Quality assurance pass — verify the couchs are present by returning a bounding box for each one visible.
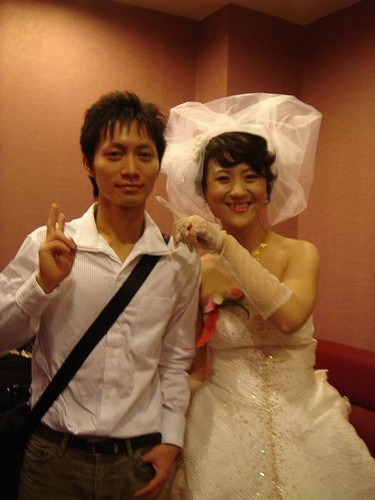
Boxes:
[314,339,375,456]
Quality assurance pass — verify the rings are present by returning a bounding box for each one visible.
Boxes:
[186,222,193,231]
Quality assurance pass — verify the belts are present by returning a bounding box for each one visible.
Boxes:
[32,423,162,455]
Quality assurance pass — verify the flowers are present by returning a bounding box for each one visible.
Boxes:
[194,287,250,347]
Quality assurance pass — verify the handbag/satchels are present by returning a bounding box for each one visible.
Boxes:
[0,352,32,500]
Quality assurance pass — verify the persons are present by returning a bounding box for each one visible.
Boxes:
[0,89,203,500]
[156,131,375,500]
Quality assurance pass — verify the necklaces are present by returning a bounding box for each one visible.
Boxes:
[251,230,272,261]
